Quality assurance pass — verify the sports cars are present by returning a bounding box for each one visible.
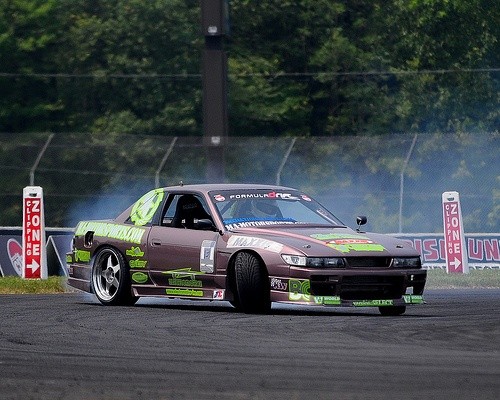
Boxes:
[67,182,427,316]
